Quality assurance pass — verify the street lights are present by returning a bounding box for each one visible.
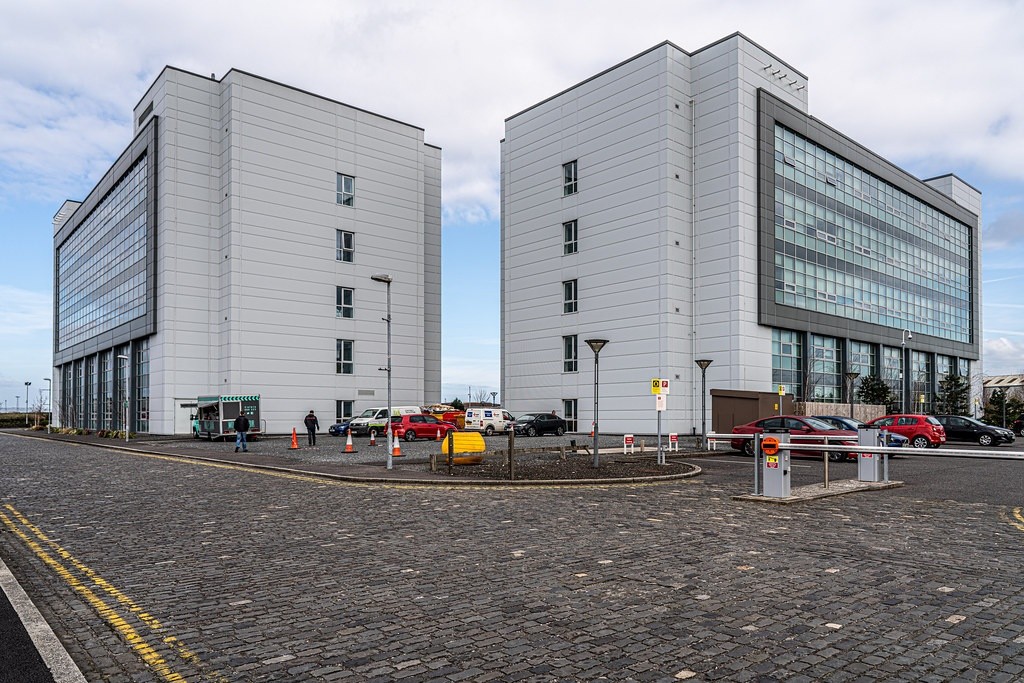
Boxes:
[371,274,394,469]
[117,354,129,442]
[695,360,713,450]
[584,340,609,469]
[15,378,51,434]
[491,392,498,407]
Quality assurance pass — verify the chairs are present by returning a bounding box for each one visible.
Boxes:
[795,422,802,430]
[899,419,904,425]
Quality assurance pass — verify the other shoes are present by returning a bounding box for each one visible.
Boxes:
[244,449,248,452]
[235,447,239,453]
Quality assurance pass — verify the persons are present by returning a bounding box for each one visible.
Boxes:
[234,411,250,453]
[304,410,319,446]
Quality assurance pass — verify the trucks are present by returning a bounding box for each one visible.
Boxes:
[190,394,261,442]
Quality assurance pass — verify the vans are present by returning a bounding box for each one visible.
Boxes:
[349,406,421,438]
[464,409,515,437]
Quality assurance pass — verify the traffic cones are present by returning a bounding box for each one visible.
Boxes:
[341,429,377,454]
[287,427,300,450]
[392,430,406,457]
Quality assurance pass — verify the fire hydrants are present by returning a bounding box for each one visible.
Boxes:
[901,329,913,414]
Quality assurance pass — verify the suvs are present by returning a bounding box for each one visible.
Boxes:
[384,414,458,441]
[1014,413,1024,437]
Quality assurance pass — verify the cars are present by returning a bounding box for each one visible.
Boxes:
[731,415,859,463]
[504,413,566,437]
[329,415,358,437]
[936,415,1016,447]
[812,415,946,458]
